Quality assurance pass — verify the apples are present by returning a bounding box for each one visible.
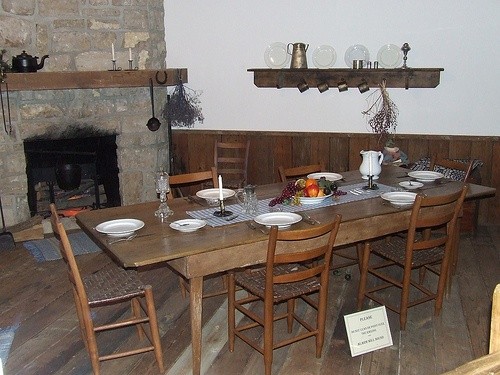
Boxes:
[302,178,324,197]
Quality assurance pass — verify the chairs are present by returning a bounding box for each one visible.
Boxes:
[164,141,474,375]
[48,203,165,375]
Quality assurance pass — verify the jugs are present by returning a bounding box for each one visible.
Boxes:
[359,150,383,180]
[235,184,258,215]
[286,43,310,69]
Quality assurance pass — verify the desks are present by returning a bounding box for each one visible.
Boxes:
[75,163,495,375]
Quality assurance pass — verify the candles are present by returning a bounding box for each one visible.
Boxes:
[112,43,115,60]
[128,47,132,60]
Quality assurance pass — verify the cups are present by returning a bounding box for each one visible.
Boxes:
[352,60,363,69]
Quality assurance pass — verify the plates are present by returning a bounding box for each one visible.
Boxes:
[195,188,235,199]
[377,44,403,69]
[95,218,145,237]
[264,42,290,69]
[307,172,343,182]
[291,190,333,204]
[169,218,207,232]
[398,180,424,190]
[344,44,369,69]
[254,212,302,229]
[408,170,444,182]
[312,45,336,69]
[381,191,417,205]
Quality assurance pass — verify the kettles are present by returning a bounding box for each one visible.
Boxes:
[11,51,49,73]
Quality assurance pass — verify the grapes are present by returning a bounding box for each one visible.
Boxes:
[335,190,347,196]
[268,182,303,206]
